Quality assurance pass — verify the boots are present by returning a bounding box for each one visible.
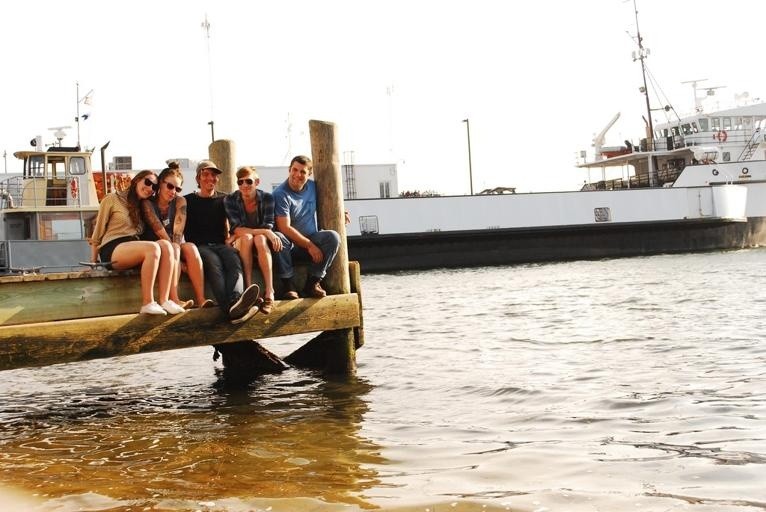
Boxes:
[303,274,327,297]
[280,279,298,300]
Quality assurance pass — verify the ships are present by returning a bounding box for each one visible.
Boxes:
[0,1,766,274]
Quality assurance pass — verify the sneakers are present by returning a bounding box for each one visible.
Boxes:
[177,300,194,309]
[161,300,185,315]
[226,283,264,324]
[199,299,215,308]
[140,302,168,316]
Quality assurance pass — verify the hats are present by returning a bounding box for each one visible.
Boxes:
[196,160,223,175]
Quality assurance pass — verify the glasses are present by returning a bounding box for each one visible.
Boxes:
[163,180,182,192]
[144,177,159,191]
[237,179,255,185]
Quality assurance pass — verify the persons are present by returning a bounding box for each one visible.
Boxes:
[181,162,262,325]
[271,155,350,300]
[140,161,214,310]
[89,171,184,316]
[223,167,282,314]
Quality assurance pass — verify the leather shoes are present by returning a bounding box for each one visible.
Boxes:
[260,297,274,314]
